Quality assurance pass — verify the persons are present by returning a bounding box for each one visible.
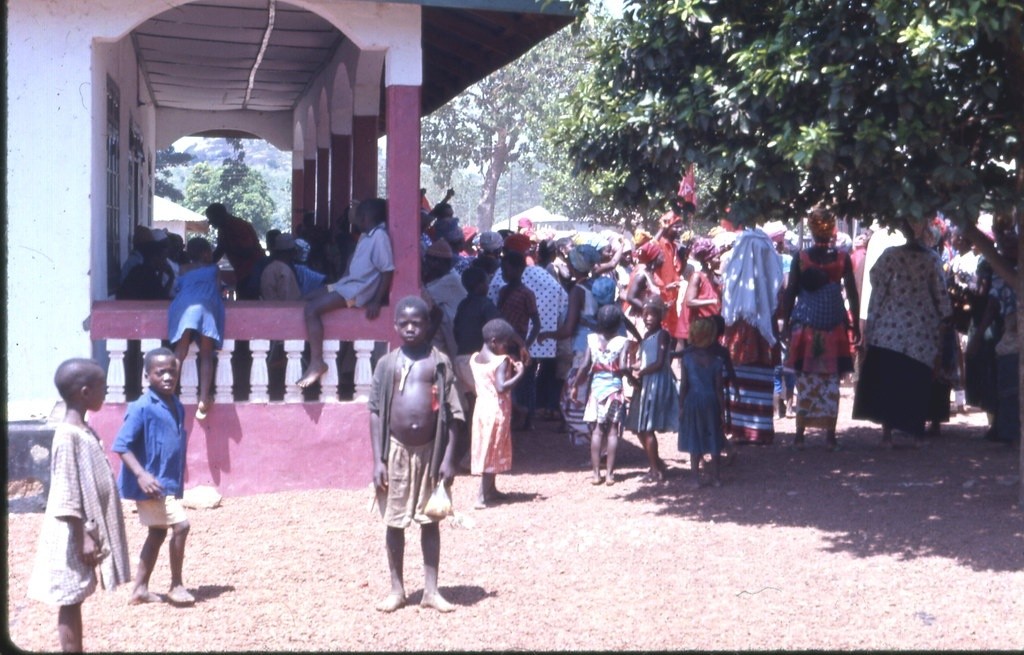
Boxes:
[630,295,673,483]
[120,197,396,384]
[167,237,227,420]
[369,295,466,614]
[420,188,1024,467]
[26,358,131,654]
[569,304,640,485]
[671,315,736,491]
[111,348,195,606]
[461,320,525,507]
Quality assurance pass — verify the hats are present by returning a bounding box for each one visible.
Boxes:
[690,317,717,348]
[643,295,665,311]
[427,237,453,258]
[592,276,616,306]
[570,245,601,273]
[807,210,836,236]
[504,234,531,255]
[693,238,718,262]
[481,232,503,252]
